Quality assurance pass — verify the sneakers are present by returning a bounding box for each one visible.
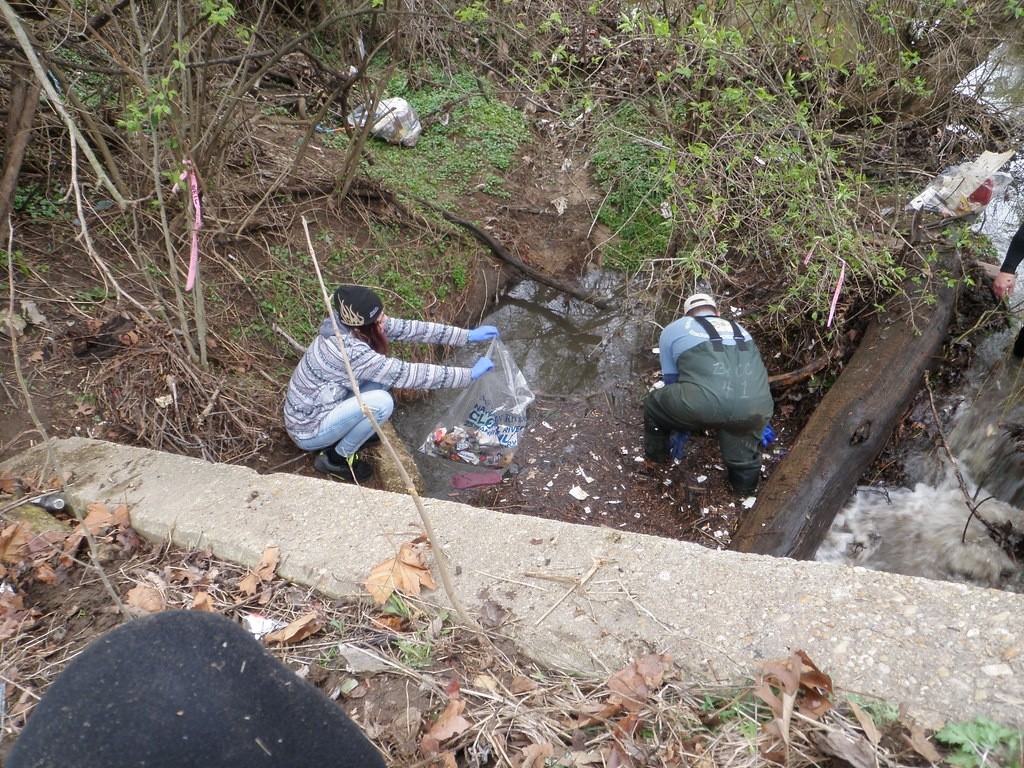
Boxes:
[366,432,380,443]
[314,449,373,483]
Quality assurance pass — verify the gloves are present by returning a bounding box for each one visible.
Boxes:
[471,357,493,380]
[467,325,500,342]
[761,424,775,447]
[664,373,690,460]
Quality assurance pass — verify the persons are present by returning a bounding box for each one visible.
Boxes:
[283,285,500,486]
[992,221,1024,360]
[642,293,774,499]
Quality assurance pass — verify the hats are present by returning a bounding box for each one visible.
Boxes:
[334,285,383,327]
[684,294,718,317]
